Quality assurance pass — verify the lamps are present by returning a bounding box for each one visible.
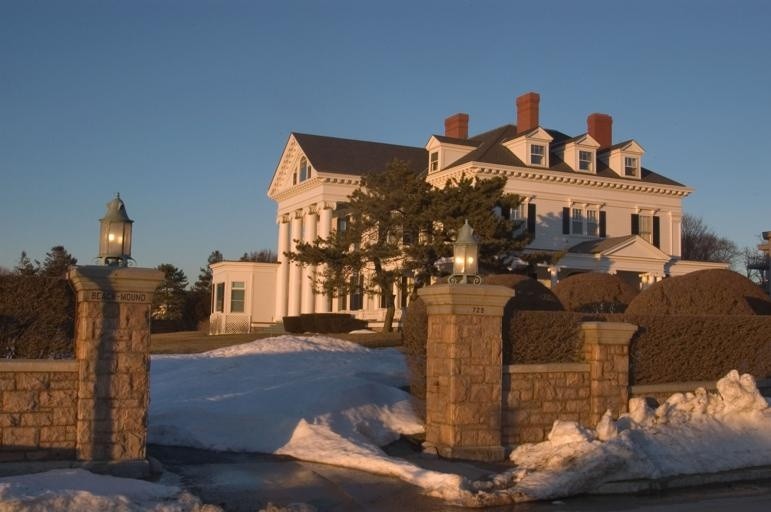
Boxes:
[95,189,137,268]
[447,220,484,287]
